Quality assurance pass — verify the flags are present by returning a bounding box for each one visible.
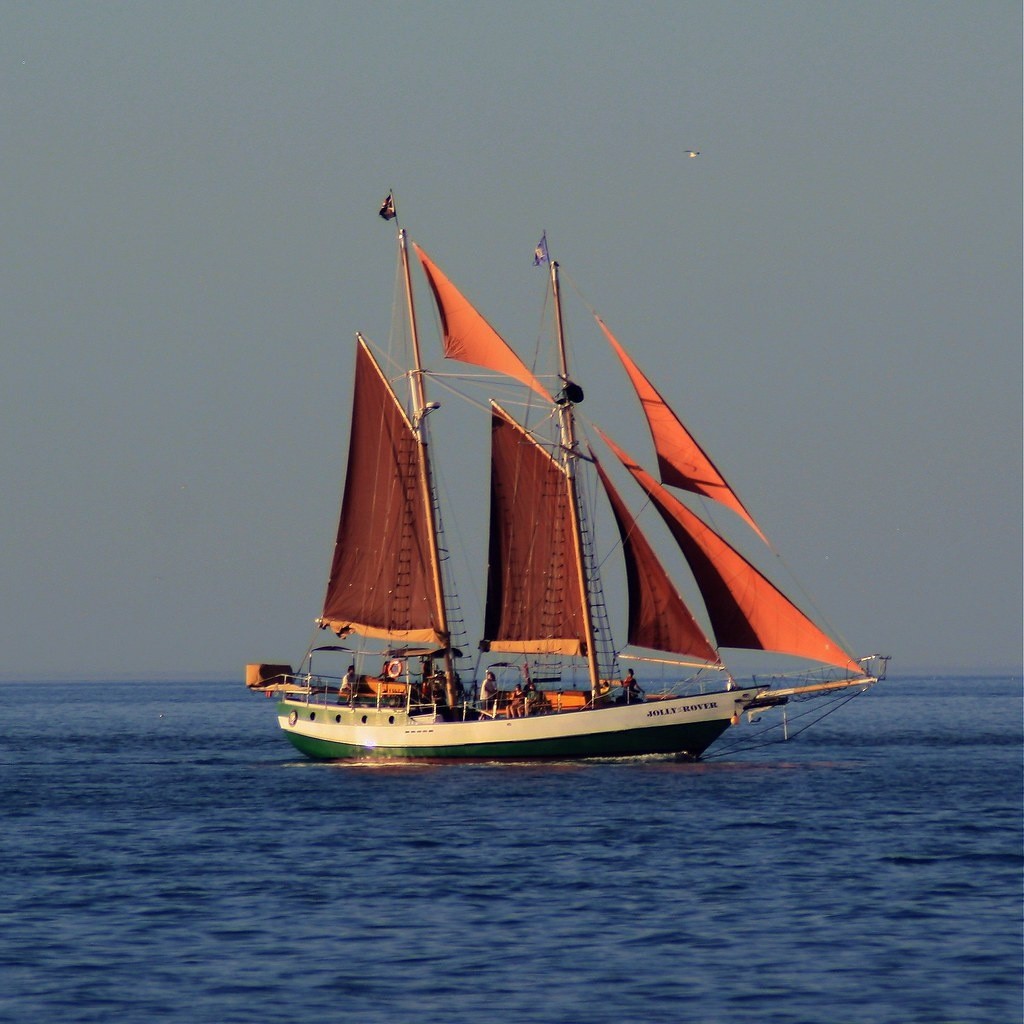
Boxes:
[378,193,398,220]
[533,235,547,266]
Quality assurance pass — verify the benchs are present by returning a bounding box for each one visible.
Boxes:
[479,707,524,719]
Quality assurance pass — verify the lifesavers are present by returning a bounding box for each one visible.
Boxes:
[387,659,403,678]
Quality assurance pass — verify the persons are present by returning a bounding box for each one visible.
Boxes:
[429,672,547,721]
[623,669,646,705]
[338,665,358,708]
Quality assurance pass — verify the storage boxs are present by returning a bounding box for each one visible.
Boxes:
[246,664,293,687]
[544,690,590,711]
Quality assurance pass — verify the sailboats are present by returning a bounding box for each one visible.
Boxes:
[243,184,888,766]
